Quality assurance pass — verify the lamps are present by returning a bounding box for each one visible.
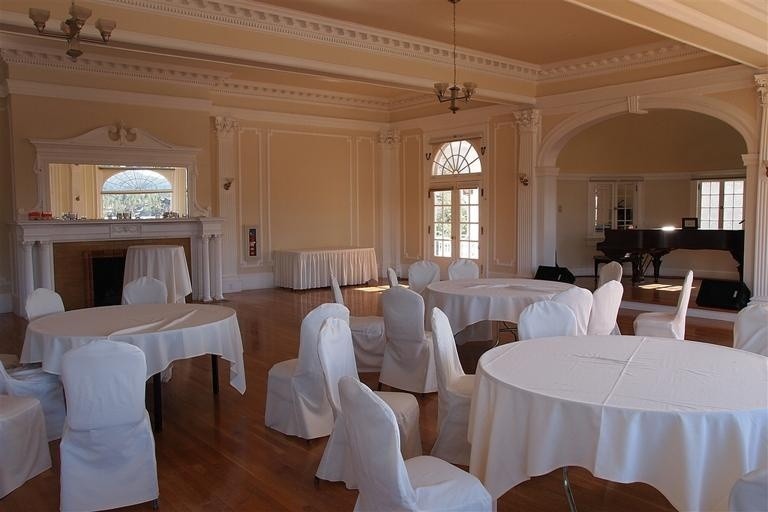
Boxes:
[223,176,235,190]
[28,0,117,63]
[431,0,478,115]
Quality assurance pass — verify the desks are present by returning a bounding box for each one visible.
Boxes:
[272,246,379,290]
[18,303,247,435]
[420,275,597,353]
[471,334,765,510]
[9,219,224,318]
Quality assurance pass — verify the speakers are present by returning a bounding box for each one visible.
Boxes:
[534,265,575,284]
[696,278,751,311]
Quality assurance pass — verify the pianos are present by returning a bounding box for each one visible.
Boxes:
[597,228,744,283]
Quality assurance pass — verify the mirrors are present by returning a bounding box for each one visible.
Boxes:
[49,163,189,220]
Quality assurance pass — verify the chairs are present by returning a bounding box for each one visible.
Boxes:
[120,275,171,305]
[428,304,481,471]
[591,278,625,336]
[336,372,493,510]
[323,260,390,379]
[383,266,399,290]
[0,393,54,500]
[731,303,767,357]
[314,316,424,490]
[405,260,443,294]
[448,258,478,282]
[264,302,352,444]
[56,340,164,512]
[24,287,67,321]
[375,282,439,402]
[547,284,595,337]
[1,355,67,446]
[514,299,579,340]
[631,267,697,342]
[598,260,625,336]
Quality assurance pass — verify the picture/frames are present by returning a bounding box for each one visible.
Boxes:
[682,217,698,230]
